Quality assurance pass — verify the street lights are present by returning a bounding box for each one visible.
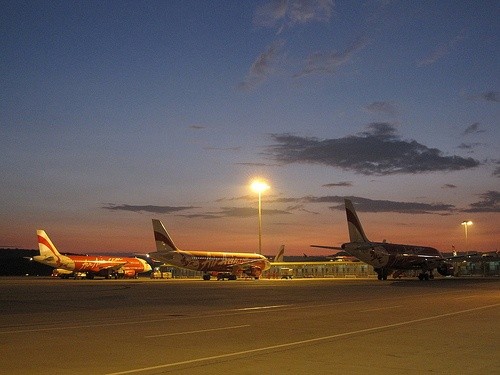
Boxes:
[250,179,267,254]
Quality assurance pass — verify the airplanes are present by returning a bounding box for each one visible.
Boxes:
[309,197,445,281]
[23,229,153,280]
[147,219,271,280]
